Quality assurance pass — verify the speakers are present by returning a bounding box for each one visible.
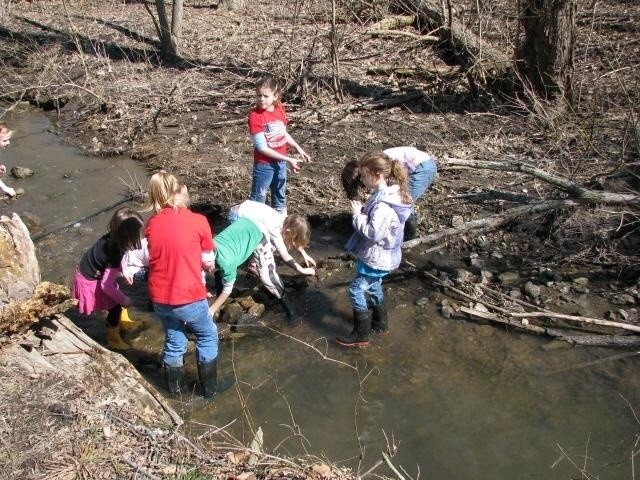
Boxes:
[197,355,236,398]
[161,358,197,394]
[278,288,304,330]
[403,214,419,244]
[119,307,143,330]
[104,324,131,351]
[335,303,390,348]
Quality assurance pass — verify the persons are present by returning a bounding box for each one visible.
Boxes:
[224,199,319,277]
[142,169,238,399]
[70,206,146,352]
[207,217,301,329]
[245,74,312,217]
[339,144,438,241]
[119,236,217,300]
[335,149,417,350]
